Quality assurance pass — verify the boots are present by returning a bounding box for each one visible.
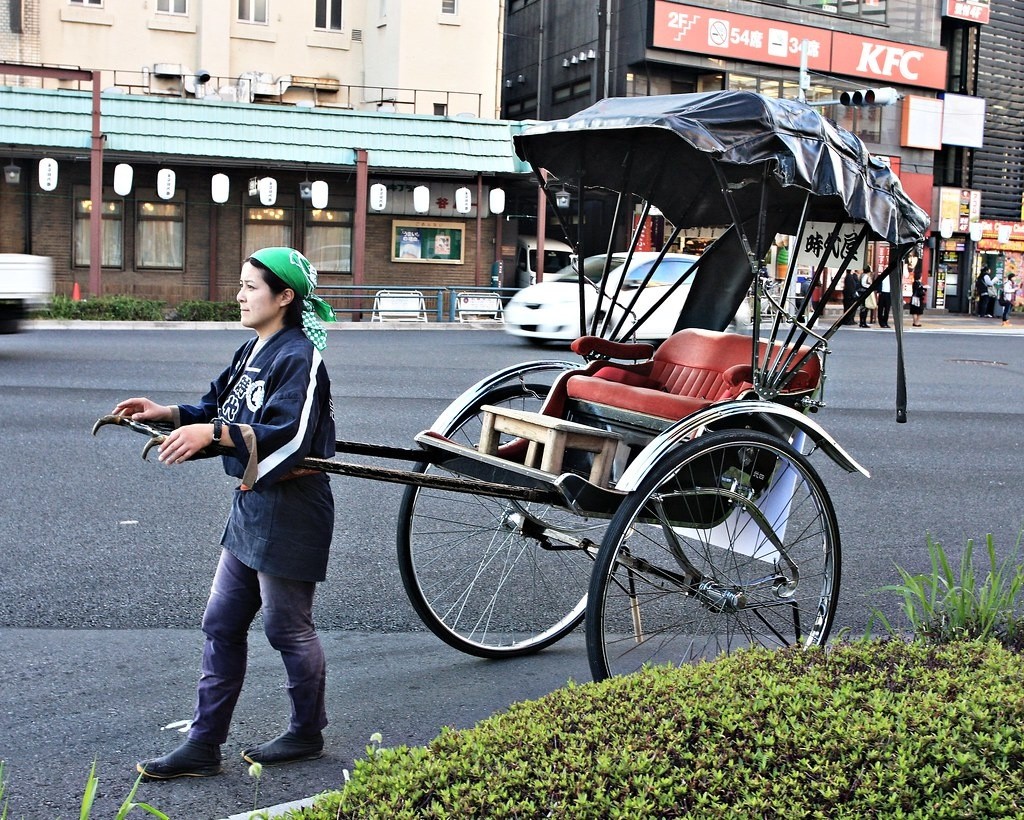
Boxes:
[859,311,870,327]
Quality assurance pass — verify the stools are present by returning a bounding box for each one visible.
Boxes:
[478,405,623,488]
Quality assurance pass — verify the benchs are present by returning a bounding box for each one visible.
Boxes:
[565,327,820,432]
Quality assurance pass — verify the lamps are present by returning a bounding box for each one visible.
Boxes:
[555,184,570,208]
[247,164,262,197]
[299,162,313,200]
[4,146,21,184]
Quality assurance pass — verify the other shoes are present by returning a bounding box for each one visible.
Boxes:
[242,730,324,766]
[913,323,921,326]
[880,323,890,328]
[137,738,224,778]
[984,314,992,317]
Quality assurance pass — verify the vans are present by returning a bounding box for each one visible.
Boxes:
[503,251,751,344]
[515,235,579,291]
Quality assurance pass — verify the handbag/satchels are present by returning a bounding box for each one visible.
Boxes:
[988,287,997,298]
[865,291,877,309]
[912,297,920,307]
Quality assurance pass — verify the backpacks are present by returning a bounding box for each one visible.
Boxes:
[998,282,1007,306]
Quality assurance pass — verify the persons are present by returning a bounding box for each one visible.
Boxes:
[112,246,337,776]
[811,280,825,318]
[910,271,928,326]
[905,244,920,272]
[843,265,891,328]
[976,266,1018,327]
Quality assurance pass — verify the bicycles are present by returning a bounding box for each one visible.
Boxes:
[739,281,798,326]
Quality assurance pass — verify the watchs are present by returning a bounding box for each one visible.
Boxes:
[209,418,222,445]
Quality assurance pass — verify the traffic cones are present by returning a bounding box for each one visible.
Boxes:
[72,283,80,300]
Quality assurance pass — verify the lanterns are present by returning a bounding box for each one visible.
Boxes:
[970,222,983,242]
[157,169,176,199]
[489,188,505,215]
[212,173,229,205]
[39,158,58,191]
[370,183,387,212]
[259,177,277,205]
[997,224,1012,245]
[455,187,472,214]
[114,163,133,197]
[940,218,954,239]
[311,181,328,209]
[413,185,429,214]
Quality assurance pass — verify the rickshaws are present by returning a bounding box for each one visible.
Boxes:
[92,91,928,685]
